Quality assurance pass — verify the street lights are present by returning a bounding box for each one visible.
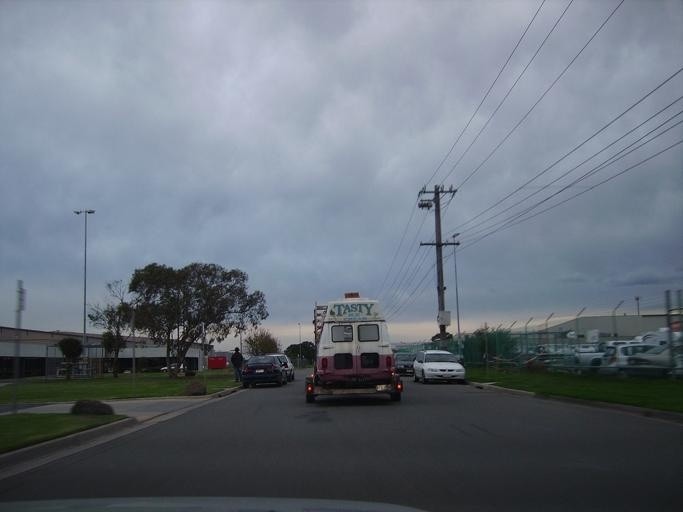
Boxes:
[297,322,301,368]
[452,233,463,364]
[631,297,640,317]
[73,209,94,376]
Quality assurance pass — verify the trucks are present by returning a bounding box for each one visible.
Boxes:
[207,356,229,369]
[304,292,402,401]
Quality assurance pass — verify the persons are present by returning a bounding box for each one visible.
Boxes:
[230,347,245,381]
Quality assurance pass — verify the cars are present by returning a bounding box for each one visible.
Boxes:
[413,350,466,384]
[263,353,294,382]
[393,352,415,377]
[510,327,683,380]
[159,363,187,372]
[240,355,287,388]
